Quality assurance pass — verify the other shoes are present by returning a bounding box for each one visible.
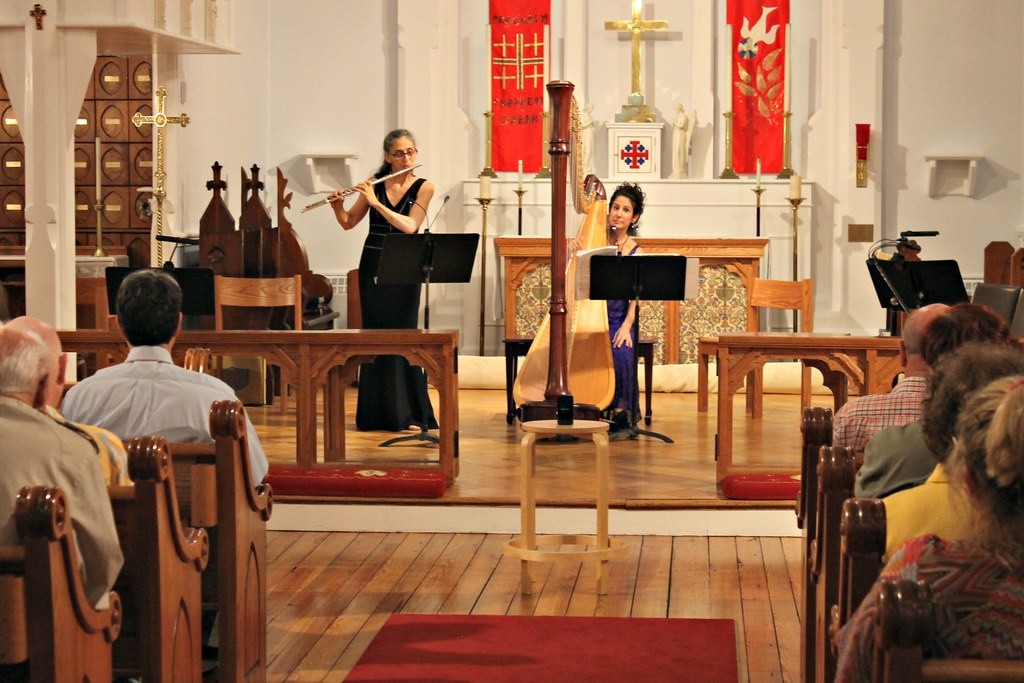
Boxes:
[611,413,631,432]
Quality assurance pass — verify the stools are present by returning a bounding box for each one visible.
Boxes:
[501,419,632,596]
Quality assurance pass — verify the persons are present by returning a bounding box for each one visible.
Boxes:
[0,316,135,612]
[60,271,269,488]
[833,303,1024,683]
[567,181,647,432]
[330,128,440,432]
[668,103,689,177]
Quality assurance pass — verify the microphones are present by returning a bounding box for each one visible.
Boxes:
[901,231,939,237]
[157,235,200,245]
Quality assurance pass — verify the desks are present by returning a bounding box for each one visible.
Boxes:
[0,254,129,319]
[715,329,906,486]
[55,328,461,489]
[492,237,770,364]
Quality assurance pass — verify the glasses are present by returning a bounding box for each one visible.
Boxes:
[388,148,417,158]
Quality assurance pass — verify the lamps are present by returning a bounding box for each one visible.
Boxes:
[855,122,872,188]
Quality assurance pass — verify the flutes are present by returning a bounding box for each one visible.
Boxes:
[299,162,423,214]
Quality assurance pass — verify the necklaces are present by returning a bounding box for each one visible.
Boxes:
[608,234,629,255]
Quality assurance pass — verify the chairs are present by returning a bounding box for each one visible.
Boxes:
[696,277,814,420]
[971,282,1020,325]
[1010,287,1024,336]
[214,274,302,417]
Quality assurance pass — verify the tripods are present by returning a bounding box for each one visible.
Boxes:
[589,255,687,444]
[379,233,480,447]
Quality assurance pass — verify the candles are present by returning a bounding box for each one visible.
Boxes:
[789,172,802,199]
[542,24,551,112]
[95,135,102,200]
[725,23,733,113]
[756,158,761,190]
[518,159,523,191]
[485,23,493,112]
[479,175,491,198]
[783,21,792,115]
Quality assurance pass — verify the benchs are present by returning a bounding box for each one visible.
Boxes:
[828,497,887,659]
[123,400,274,683]
[866,577,1024,683]
[807,443,856,683]
[501,333,658,426]
[795,406,863,683]
[107,434,210,683]
[0,485,122,683]
